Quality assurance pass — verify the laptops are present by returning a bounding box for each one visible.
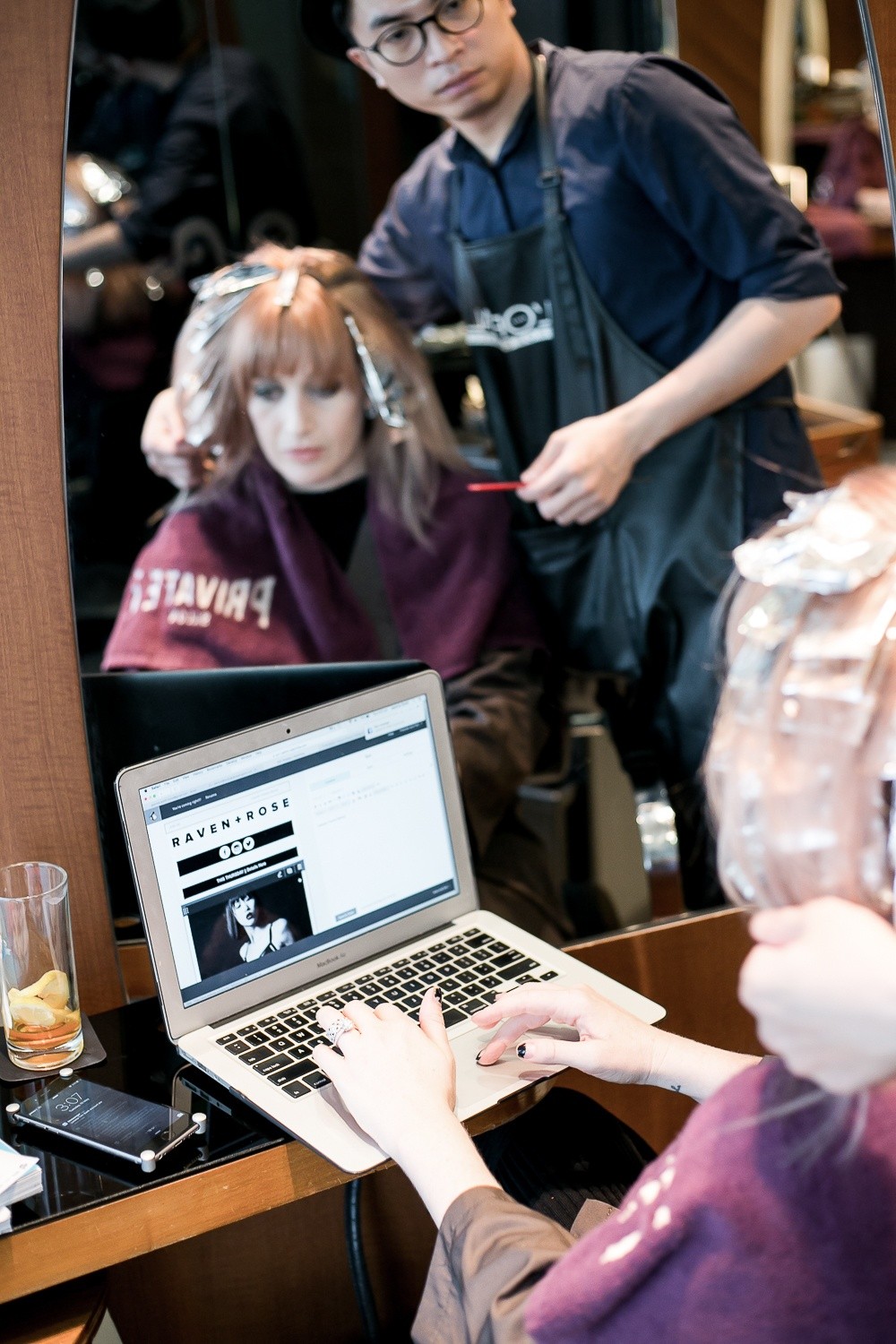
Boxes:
[116,671,670,1174]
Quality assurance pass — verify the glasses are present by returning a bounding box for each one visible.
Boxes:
[356,0,486,68]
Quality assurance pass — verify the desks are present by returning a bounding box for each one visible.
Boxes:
[0,997,560,1344]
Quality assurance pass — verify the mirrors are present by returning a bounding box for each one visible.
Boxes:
[0,0,896,1013]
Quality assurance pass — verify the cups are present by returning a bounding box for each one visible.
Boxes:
[1,860,84,1072]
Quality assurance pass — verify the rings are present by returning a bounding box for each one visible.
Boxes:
[328,1018,358,1047]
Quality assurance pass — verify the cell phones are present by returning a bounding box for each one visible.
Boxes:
[14,1075,200,1164]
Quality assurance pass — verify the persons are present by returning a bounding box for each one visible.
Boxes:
[313,466,896,1344]
[59,0,302,283]
[100,242,571,947]
[813,51,893,228]
[343,0,845,915]
[225,891,295,962]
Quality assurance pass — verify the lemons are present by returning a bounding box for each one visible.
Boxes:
[9,996,55,1027]
